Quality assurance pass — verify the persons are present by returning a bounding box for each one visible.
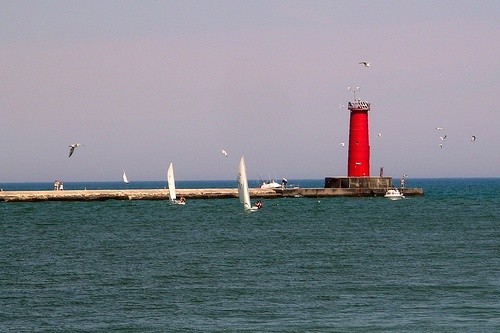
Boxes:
[177,196,184,202]
[399,176,405,188]
[54,180,64,191]
[255,199,261,207]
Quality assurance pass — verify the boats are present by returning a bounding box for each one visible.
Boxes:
[260,179,282,189]
[384,190,405,200]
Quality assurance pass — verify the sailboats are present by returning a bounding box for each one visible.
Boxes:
[167,161,187,205]
[237,155,259,213]
[122,168,130,184]
